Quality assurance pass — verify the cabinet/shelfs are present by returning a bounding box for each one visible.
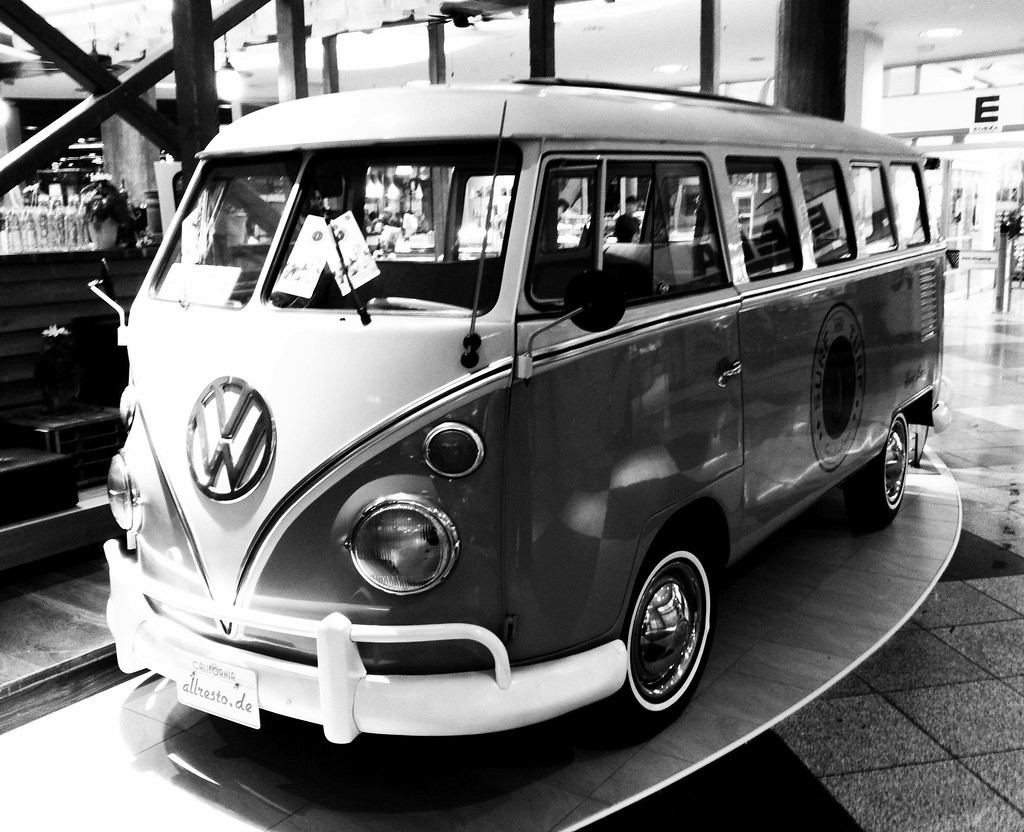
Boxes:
[34,141,104,175]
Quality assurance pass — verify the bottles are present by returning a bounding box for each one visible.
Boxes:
[0,173,160,255]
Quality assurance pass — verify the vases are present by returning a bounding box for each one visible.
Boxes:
[88,217,119,251]
[35,339,81,417]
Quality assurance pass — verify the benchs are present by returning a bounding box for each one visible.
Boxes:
[531,246,598,302]
[356,260,504,311]
[606,243,722,290]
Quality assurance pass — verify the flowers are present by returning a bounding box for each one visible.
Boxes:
[41,322,71,338]
[78,171,134,233]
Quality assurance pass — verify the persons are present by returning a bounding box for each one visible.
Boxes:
[613,196,639,243]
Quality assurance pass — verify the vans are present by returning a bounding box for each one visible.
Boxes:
[81,74,949,743]
